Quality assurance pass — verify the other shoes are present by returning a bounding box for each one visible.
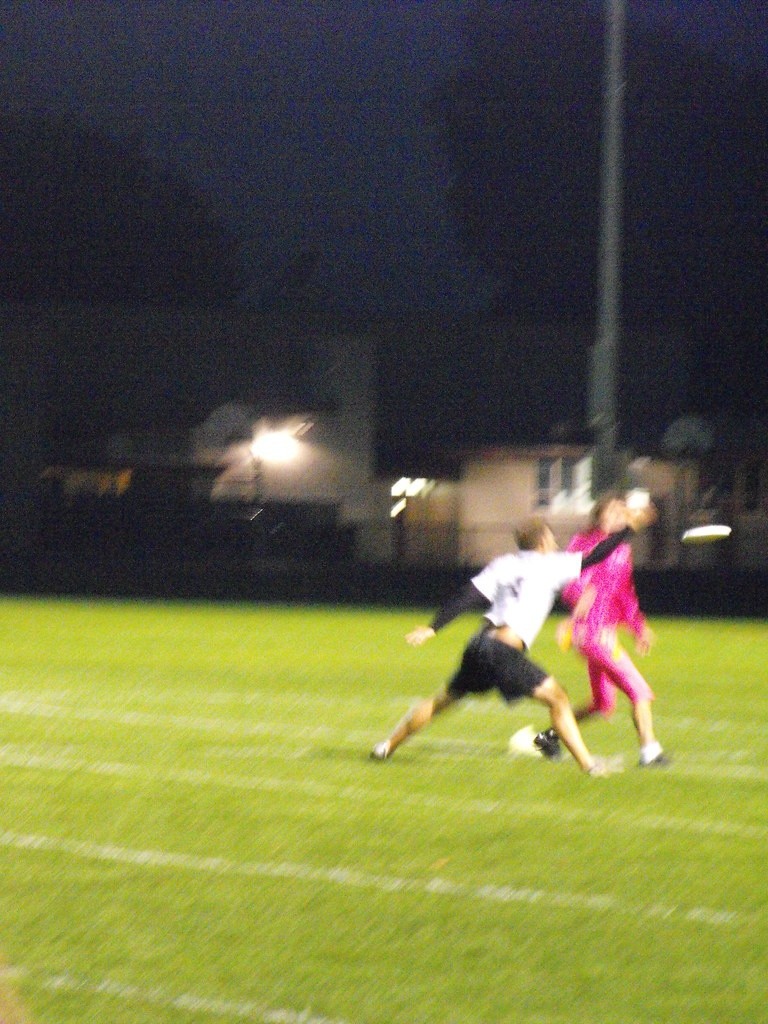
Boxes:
[587,756,621,778]
[534,731,562,764]
[638,751,674,769]
[371,741,390,761]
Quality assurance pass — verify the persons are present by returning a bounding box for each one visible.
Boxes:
[369,498,659,774]
[252,462,265,504]
[534,491,671,768]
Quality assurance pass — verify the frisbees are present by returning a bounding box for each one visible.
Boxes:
[682,524,732,542]
